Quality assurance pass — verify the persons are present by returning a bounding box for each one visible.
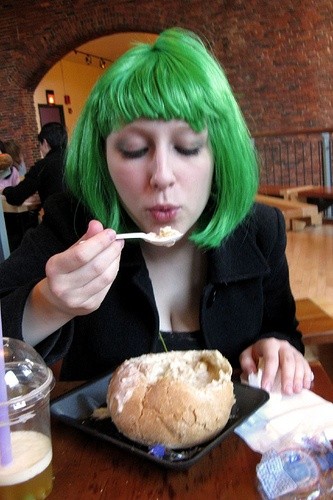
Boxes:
[0,139,29,194]
[2,122,86,245]
[0,27,315,397]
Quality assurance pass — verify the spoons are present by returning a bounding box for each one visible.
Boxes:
[80,228,183,247]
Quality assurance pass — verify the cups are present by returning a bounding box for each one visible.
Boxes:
[0,335,56,500]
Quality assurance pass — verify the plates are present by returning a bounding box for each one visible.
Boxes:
[49,359,270,473]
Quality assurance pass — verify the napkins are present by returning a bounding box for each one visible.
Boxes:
[234,365,333,455]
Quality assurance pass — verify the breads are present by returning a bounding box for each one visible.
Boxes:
[107,350,236,451]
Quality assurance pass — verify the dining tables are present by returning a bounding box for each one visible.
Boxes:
[44,361,333,500]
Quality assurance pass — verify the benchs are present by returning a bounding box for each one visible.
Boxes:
[295,298,333,382]
